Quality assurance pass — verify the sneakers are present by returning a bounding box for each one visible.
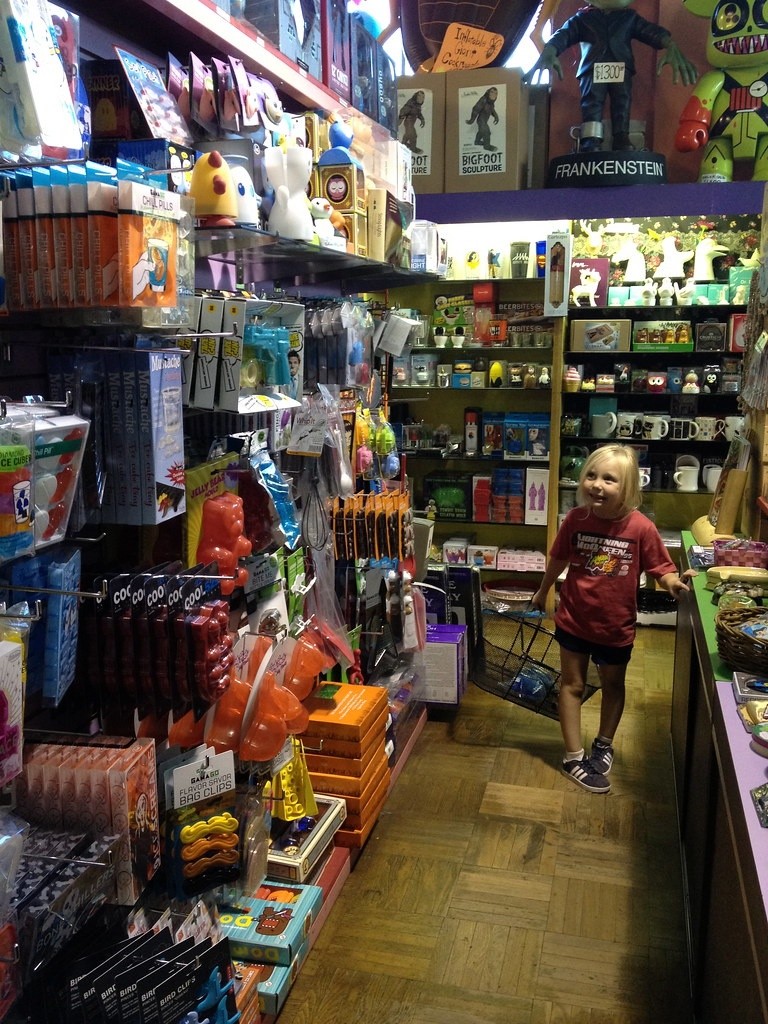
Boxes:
[589,738,614,777]
[561,752,611,793]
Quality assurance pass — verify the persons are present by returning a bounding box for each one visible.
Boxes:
[521,0,701,154]
[530,445,699,792]
[280,350,303,404]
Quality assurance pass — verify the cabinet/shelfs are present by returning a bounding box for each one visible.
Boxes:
[672,531,768,1024]
[381,178,766,626]
[0,0,768,1024]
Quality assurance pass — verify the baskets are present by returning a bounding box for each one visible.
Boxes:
[714,606,768,678]
[469,588,602,722]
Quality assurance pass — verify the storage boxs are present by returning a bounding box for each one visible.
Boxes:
[422,469,473,522]
[394,72,444,194]
[497,547,545,564]
[0,157,442,1024]
[424,623,467,693]
[442,540,467,564]
[411,632,462,704]
[524,467,549,525]
[467,544,498,569]
[496,561,546,572]
[471,473,492,523]
[443,67,527,192]
[483,408,550,462]
[567,251,755,351]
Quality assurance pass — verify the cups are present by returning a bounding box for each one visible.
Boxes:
[559,490,575,512]
[629,445,648,463]
[644,414,670,439]
[694,417,724,441]
[675,455,701,470]
[451,336,465,348]
[413,315,430,346]
[720,417,746,441]
[639,468,652,491]
[674,466,700,492]
[593,412,617,438]
[435,335,448,348]
[669,418,698,440]
[707,468,722,492]
[703,465,721,484]
[617,411,644,439]
